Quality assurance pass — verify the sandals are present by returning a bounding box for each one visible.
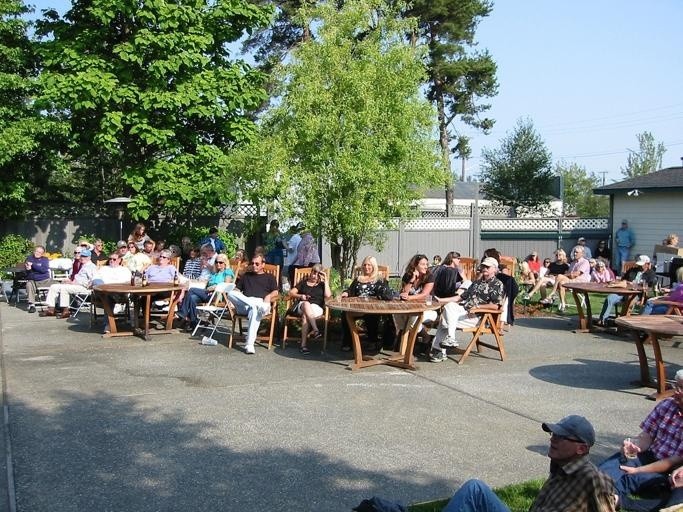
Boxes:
[313,330,322,339]
[299,346,311,355]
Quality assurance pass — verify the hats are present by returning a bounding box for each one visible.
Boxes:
[579,237,586,242]
[531,252,538,256]
[81,250,91,257]
[622,219,628,224]
[117,240,127,249]
[481,257,499,268]
[541,414,596,447]
[635,255,651,267]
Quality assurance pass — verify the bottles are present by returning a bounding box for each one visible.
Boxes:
[130,270,146,287]
[173,270,178,287]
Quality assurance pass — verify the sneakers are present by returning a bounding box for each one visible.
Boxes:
[60,310,71,318]
[260,304,271,316]
[440,336,460,348]
[246,345,256,354]
[594,319,608,326]
[28,304,36,313]
[341,339,378,352]
[430,352,447,363]
[39,309,56,317]
[104,325,111,333]
[523,294,567,312]
[167,314,199,332]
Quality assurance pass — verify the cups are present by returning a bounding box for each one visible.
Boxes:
[615,276,621,283]
[623,438,639,458]
[336,294,342,302]
[425,295,432,305]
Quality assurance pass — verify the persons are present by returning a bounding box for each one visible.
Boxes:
[38,237,180,334]
[162,245,330,355]
[614,219,639,272]
[610,465,683,511]
[281,226,301,289]
[599,368,683,493]
[340,247,506,363]
[517,234,682,340]
[23,246,60,312]
[440,414,614,511]
[287,222,320,268]
[199,228,227,254]
[127,220,156,256]
[263,219,284,270]
